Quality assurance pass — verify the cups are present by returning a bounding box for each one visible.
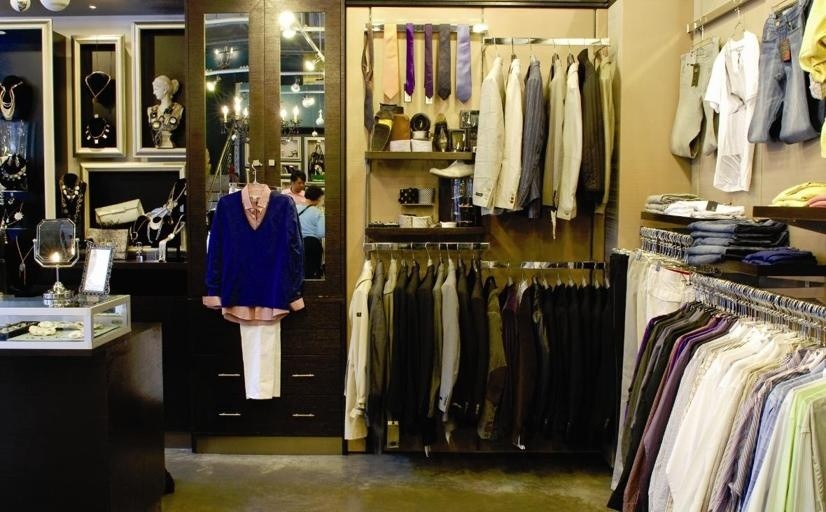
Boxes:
[398,186,434,205]
[399,215,432,228]
[440,221,457,228]
[461,206,474,227]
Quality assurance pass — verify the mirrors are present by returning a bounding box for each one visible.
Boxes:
[78,240,115,303]
[279,11,325,281]
[32,217,81,305]
[204,12,250,254]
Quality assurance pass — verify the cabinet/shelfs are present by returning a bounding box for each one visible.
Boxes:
[1,322,165,512]
[0,18,67,296]
[0,294,131,350]
[364,150,485,240]
[185,2,348,455]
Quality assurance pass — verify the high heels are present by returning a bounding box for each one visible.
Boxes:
[314,165,325,176]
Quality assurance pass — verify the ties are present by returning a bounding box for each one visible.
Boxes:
[405,23,415,96]
[438,23,451,101]
[455,23,472,104]
[423,23,434,98]
[382,24,399,99]
[361,20,375,133]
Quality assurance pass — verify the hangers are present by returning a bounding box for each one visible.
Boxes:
[727,7,743,38]
[638,225,719,276]
[679,270,826,356]
[486,261,612,288]
[491,34,610,63]
[770,1,797,22]
[685,16,712,50]
[248,162,264,200]
[362,242,481,271]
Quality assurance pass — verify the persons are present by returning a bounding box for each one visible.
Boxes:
[147,75,183,149]
[279,170,326,280]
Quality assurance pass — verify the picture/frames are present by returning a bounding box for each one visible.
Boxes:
[281,162,301,184]
[80,162,187,259]
[280,136,302,162]
[303,137,325,187]
[130,19,186,159]
[70,34,126,160]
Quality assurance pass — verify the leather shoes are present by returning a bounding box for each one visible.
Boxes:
[429,159,474,179]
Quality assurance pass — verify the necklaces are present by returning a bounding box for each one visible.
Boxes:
[85,118,110,145]
[0,81,24,121]
[149,103,174,148]
[15,235,34,285]
[1,163,27,181]
[0,202,24,244]
[85,70,111,104]
[59,175,84,225]
[129,181,188,259]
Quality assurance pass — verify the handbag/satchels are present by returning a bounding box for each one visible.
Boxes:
[308,143,325,173]
[84,227,130,260]
[95,198,147,226]
